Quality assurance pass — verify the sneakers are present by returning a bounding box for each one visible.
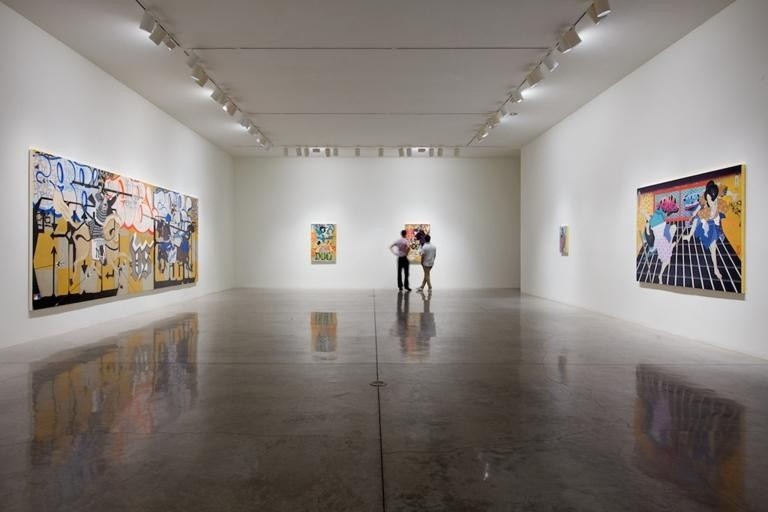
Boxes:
[399,287,432,293]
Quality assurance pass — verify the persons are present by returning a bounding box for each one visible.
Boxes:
[415,290,437,346]
[395,291,411,351]
[416,235,436,291]
[390,230,412,291]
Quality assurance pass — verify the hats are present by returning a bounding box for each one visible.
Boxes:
[648,214,665,229]
[704,180,719,203]
